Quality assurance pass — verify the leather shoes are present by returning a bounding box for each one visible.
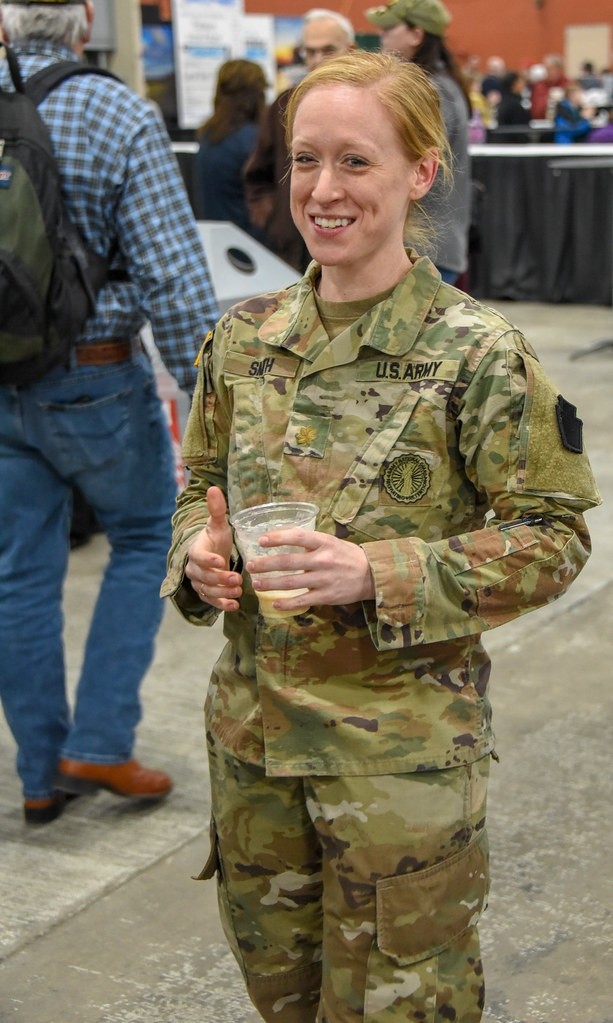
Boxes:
[53,758,172,802]
[25,793,77,821]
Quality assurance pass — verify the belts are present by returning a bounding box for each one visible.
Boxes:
[74,339,142,365]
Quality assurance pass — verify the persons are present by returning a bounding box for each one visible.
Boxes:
[463,55,613,145]
[0,0,220,824]
[366,0,470,284]
[160,53,603,1022]
[196,10,353,276]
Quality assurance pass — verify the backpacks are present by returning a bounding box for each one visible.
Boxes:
[0,43,126,386]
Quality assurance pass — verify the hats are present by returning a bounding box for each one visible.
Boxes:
[366,0,450,38]
[219,60,271,92]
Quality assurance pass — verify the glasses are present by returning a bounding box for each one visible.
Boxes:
[299,44,336,59]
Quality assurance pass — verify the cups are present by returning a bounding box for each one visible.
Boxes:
[230,502,320,620]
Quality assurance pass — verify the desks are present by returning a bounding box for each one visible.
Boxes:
[453,126,613,307]
